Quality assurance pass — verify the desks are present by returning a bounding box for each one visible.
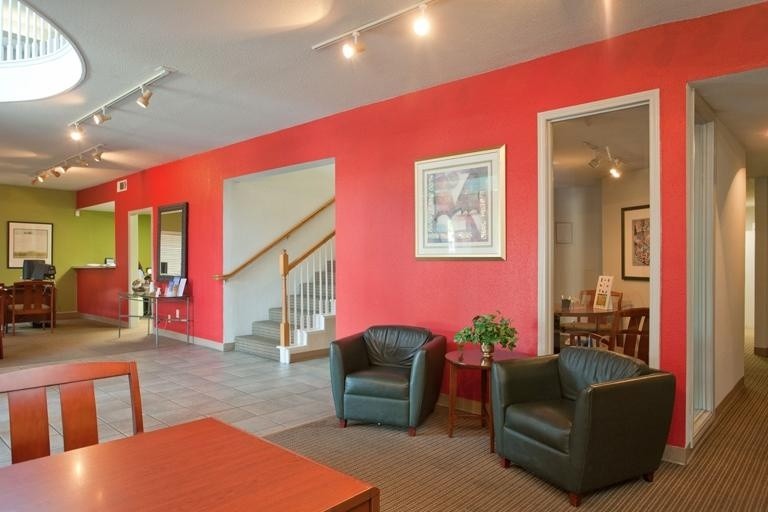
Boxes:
[5,286,56,327]
[555,303,618,347]
[118,292,190,348]
[0,416,380,512]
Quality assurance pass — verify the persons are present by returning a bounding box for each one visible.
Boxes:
[139,262,146,284]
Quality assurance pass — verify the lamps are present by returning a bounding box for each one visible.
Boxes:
[31,143,105,186]
[312,0,431,60]
[582,140,626,178]
[67,70,171,141]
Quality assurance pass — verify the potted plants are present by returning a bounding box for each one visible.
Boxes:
[453,310,520,357]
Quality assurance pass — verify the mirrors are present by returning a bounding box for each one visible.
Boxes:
[156,202,187,283]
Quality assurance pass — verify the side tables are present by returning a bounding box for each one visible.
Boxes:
[444,350,530,455]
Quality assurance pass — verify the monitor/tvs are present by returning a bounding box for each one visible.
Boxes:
[105,257,114,264]
[22,259,57,281]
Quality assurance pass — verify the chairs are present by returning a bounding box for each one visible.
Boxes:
[490,347,675,507]
[330,326,446,437]
[0,361,143,465]
[555,290,618,347]
[570,308,649,365]
[6,282,55,336]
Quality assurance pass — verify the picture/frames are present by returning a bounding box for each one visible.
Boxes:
[621,205,650,281]
[7,221,53,268]
[413,143,507,262]
[554,222,573,245]
